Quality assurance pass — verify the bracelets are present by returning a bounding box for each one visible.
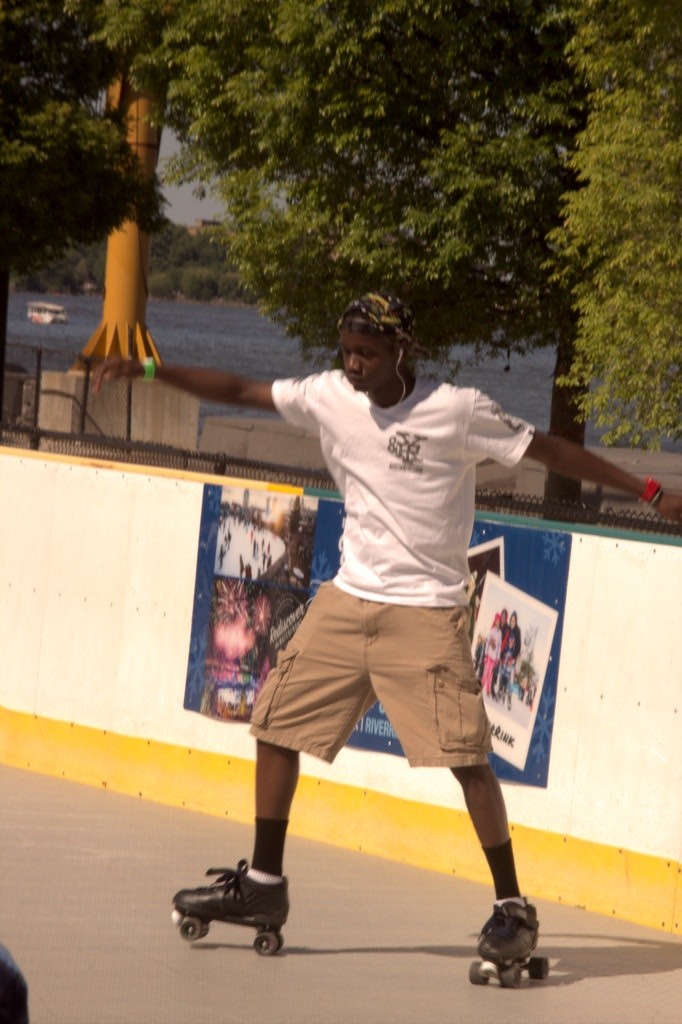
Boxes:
[140,358,152,381]
[641,479,661,509]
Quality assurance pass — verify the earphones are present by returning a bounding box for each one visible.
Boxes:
[398,347,404,366]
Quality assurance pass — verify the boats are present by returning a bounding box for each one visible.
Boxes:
[25,301,68,325]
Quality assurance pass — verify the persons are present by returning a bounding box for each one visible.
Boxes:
[479,609,522,710]
[91,293,680,988]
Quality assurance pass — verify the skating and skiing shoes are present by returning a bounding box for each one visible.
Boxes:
[469,901,549,988]
[169,860,290,957]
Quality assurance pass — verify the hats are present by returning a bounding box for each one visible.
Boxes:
[338,292,417,345]
